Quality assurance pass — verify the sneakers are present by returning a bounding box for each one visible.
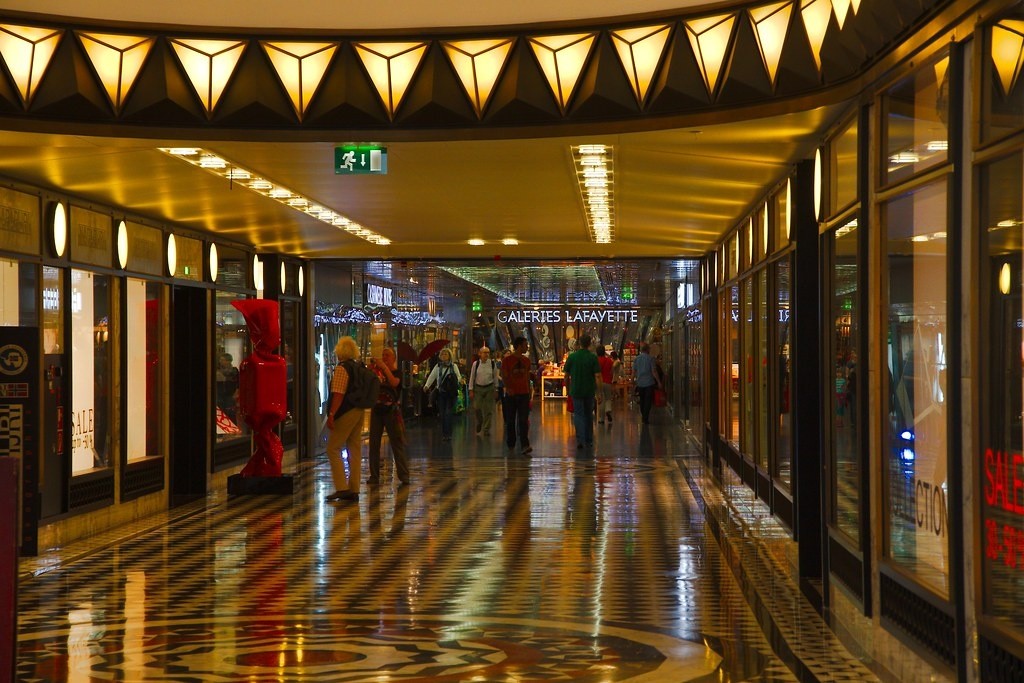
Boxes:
[508,447,514,458]
[520,445,532,454]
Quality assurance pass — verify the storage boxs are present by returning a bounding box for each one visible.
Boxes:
[623,349,638,375]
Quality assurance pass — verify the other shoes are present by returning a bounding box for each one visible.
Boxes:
[608,418,612,424]
[367,477,379,483]
[338,493,359,500]
[598,418,603,423]
[325,490,349,500]
[403,479,410,484]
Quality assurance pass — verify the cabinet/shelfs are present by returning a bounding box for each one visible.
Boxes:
[541,376,568,401]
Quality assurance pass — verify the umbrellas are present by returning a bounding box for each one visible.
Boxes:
[400,342,417,361]
[419,339,449,364]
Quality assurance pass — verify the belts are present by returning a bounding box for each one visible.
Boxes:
[476,382,493,387]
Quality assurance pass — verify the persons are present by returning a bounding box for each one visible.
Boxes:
[780,355,786,414]
[631,344,664,424]
[217,353,239,425]
[836,360,856,427]
[564,335,622,449]
[324,336,365,502]
[423,348,463,440]
[468,347,496,436]
[369,486,409,559]
[496,337,537,454]
[366,348,410,486]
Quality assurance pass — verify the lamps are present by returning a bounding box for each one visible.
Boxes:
[112,219,128,271]
[249,253,305,298]
[933,18,1024,98]
[206,241,219,283]
[45,201,68,258]
[164,232,176,277]
[701,145,825,296]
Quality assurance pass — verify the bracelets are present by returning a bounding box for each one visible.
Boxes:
[329,413,334,415]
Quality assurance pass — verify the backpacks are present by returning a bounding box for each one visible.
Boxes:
[340,360,380,409]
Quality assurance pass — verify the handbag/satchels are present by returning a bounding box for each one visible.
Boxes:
[654,389,668,407]
[429,387,439,402]
[605,399,612,412]
[567,395,574,412]
[374,379,401,415]
[454,381,465,414]
[442,373,459,401]
